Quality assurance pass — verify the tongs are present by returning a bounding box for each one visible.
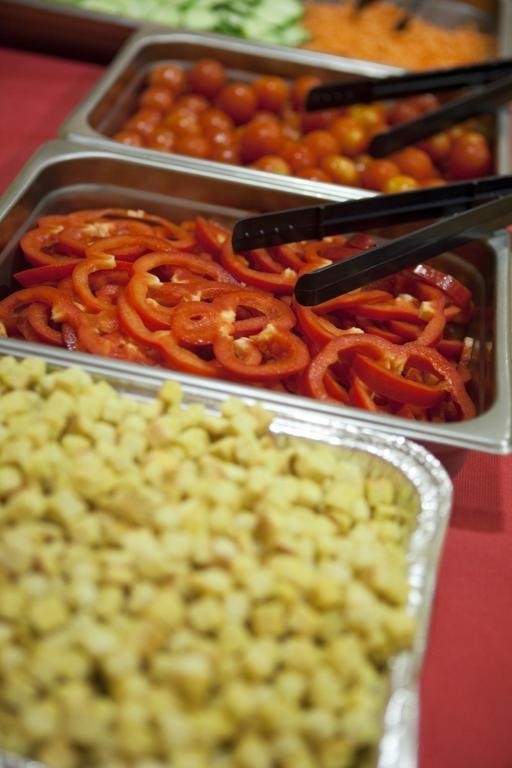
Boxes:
[304,57,512,159]
[350,0,422,30]
[230,172,512,306]
